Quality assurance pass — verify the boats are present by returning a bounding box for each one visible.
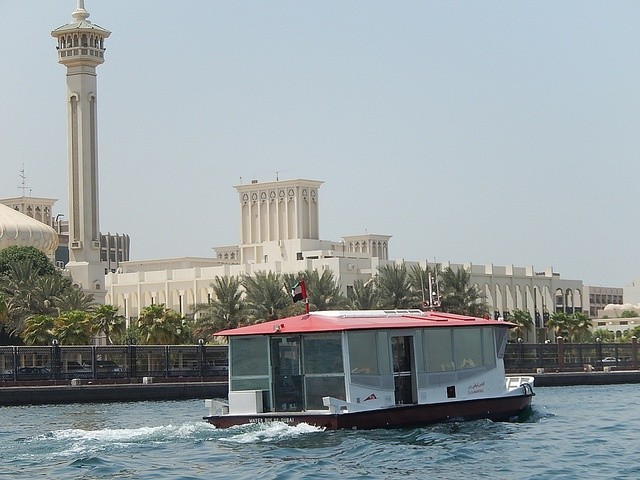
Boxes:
[202,280,535,431]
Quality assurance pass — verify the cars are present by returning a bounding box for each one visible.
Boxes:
[82,360,127,380]
[54,361,93,381]
[7,366,54,382]
[597,355,622,364]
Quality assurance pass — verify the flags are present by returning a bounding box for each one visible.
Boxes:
[292,280,309,303]
